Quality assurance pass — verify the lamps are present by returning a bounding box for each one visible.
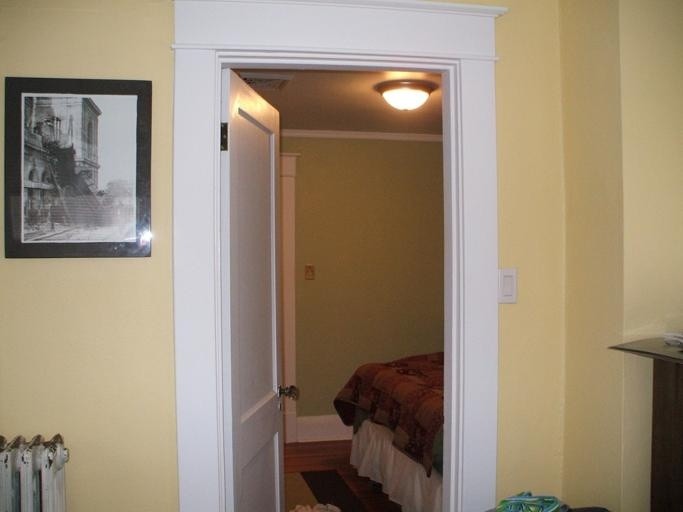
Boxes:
[372,78,439,112]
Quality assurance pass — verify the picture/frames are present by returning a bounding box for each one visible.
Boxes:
[5,77,153,257]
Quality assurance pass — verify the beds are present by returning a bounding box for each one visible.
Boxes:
[334,351,444,511]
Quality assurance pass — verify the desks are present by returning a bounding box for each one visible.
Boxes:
[608,337,683,511]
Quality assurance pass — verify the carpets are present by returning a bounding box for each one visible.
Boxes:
[286,469,369,512]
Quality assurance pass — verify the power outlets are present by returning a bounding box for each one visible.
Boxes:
[305,263,316,280]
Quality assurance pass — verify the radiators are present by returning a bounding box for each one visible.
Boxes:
[0,433,70,511]
